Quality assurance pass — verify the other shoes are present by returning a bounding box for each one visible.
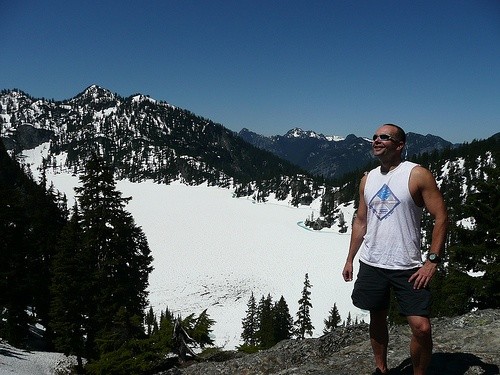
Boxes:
[371,367,389,375]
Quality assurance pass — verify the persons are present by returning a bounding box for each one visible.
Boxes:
[342,123,450,375]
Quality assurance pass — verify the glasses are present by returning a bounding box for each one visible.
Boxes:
[373,135,400,144]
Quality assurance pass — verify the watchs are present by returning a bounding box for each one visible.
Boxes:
[427,252,442,263]
